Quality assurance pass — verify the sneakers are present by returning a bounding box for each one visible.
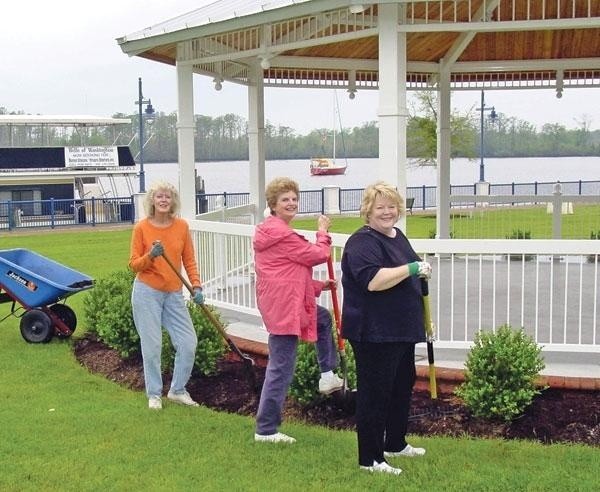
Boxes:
[149,397,163,409]
[255,432,296,444]
[384,444,427,458]
[360,460,402,475]
[319,374,344,393]
[167,389,198,407]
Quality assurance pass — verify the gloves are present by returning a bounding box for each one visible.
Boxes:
[151,243,163,257]
[190,291,204,303]
[416,261,432,281]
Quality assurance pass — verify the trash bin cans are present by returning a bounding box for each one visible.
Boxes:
[73,204,86,224]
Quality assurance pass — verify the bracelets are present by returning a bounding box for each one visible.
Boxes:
[408,262,418,276]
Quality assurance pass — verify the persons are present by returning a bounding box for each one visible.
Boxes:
[128,181,205,410]
[255,177,344,444]
[339,183,432,475]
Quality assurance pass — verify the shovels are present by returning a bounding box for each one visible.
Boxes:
[323,230,358,401]
[152,241,255,367]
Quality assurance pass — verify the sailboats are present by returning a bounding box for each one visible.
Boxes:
[308,82,351,175]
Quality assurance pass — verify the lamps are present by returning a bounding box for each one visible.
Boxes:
[553,88,564,99]
[211,76,227,91]
[346,88,359,101]
[259,55,273,70]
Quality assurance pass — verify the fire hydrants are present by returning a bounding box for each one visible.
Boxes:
[12,206,25,226]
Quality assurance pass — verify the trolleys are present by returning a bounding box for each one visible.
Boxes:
[0,248,96,346]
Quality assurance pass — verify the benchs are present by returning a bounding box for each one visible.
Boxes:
[406,197,415,216]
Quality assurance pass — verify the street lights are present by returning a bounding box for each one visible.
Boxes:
[476,90,498,183]
[133,77,160,196]
